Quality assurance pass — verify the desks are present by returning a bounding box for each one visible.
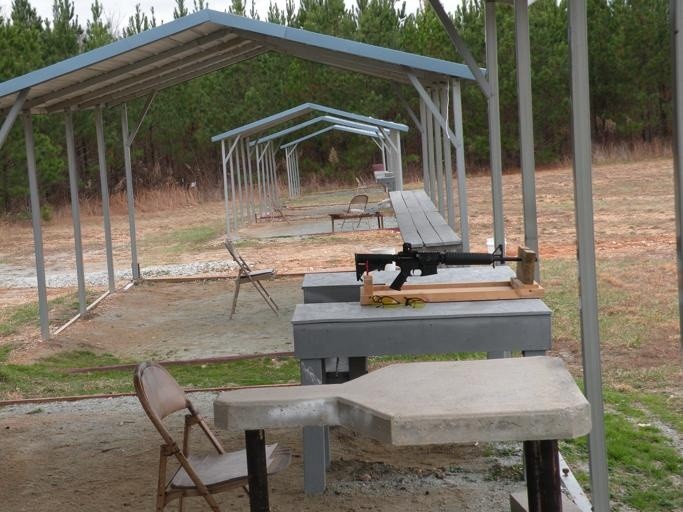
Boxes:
[374,170,396,191]
[388,188,463,253]
[329,212,384,233]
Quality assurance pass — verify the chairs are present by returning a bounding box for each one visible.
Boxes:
[222,237,280,321]
[133,361,294,512]
[340,194,368,232]
[355,175,375,193]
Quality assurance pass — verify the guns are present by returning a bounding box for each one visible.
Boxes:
[354,242,537,291]
